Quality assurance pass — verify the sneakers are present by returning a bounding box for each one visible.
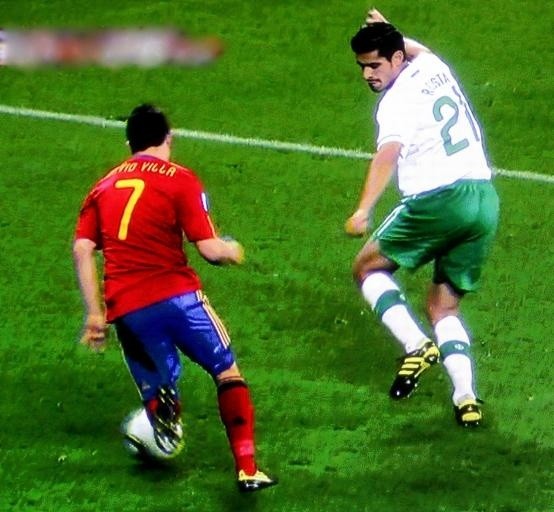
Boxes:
[388,342,441,401]
[238,469,279,493]
[153,384,184,455]
[453,398,485,427]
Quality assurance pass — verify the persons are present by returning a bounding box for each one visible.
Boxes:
[340,5,503,429]
[71,104,279,491]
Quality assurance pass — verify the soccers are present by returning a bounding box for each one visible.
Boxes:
[126,408,184,461]
